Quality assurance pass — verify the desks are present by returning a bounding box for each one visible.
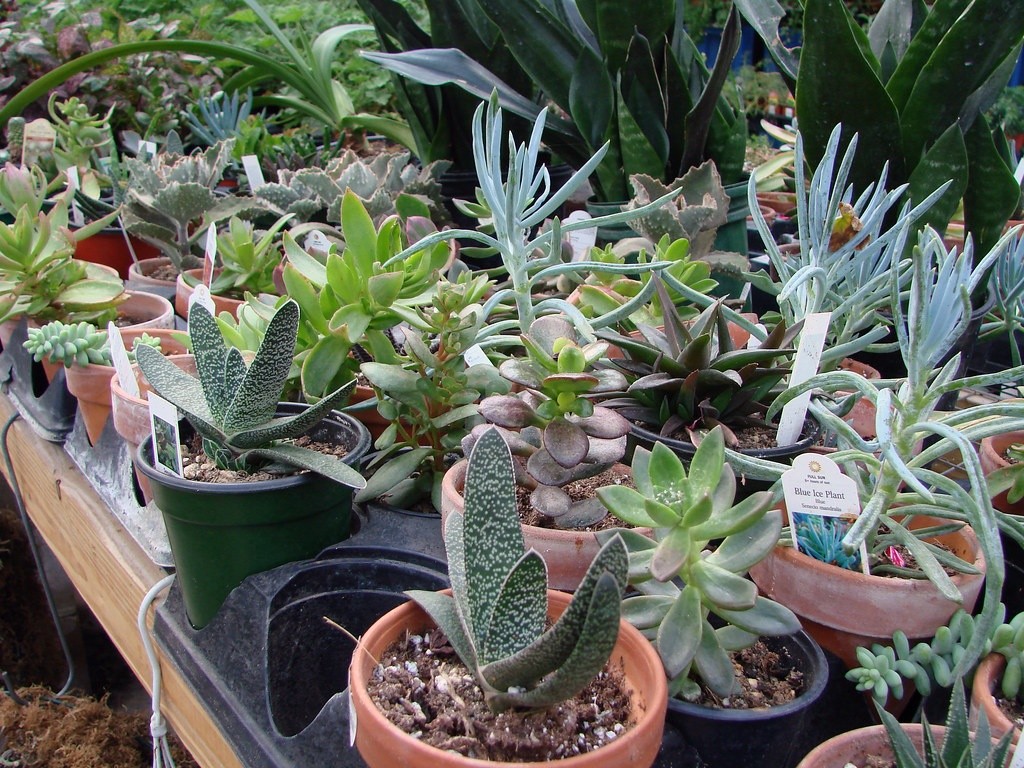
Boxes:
[0,394,244,768]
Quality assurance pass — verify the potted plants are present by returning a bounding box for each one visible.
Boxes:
[0,0,1024,768]
